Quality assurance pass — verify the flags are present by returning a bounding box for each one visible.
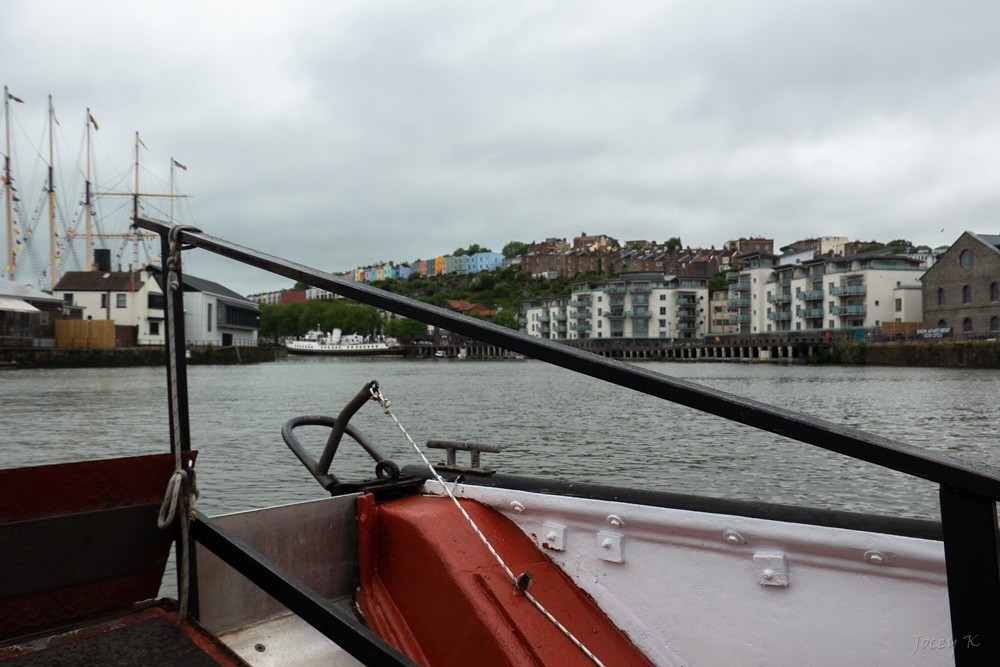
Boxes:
[7,92,186,171]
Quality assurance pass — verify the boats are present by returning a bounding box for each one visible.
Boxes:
[285,327,402,356]
[435,350,449,358]
